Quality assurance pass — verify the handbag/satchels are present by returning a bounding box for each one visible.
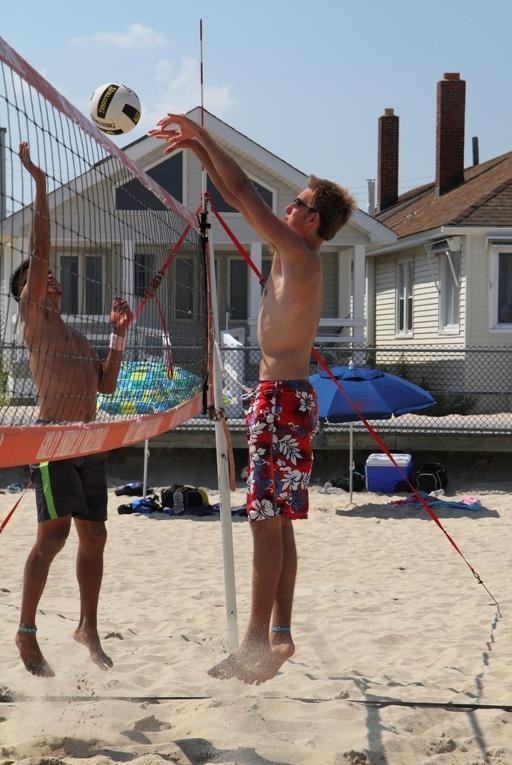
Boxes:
[161,484,210,512]
[414,461,448,492]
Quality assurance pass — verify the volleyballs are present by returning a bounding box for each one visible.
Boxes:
[90,83,141,134]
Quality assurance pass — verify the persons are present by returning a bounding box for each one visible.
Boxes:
[142,110,356,684]
[9,140,134,677]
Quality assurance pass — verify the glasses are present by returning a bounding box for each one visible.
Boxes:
[292,198,311,212]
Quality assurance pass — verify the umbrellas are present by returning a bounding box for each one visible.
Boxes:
[302,365,437,503]
[95,357,236,500]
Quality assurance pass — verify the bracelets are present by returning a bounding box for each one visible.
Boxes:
[108,332,127,353]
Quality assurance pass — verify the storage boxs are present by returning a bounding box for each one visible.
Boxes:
[365,452,415,494]
